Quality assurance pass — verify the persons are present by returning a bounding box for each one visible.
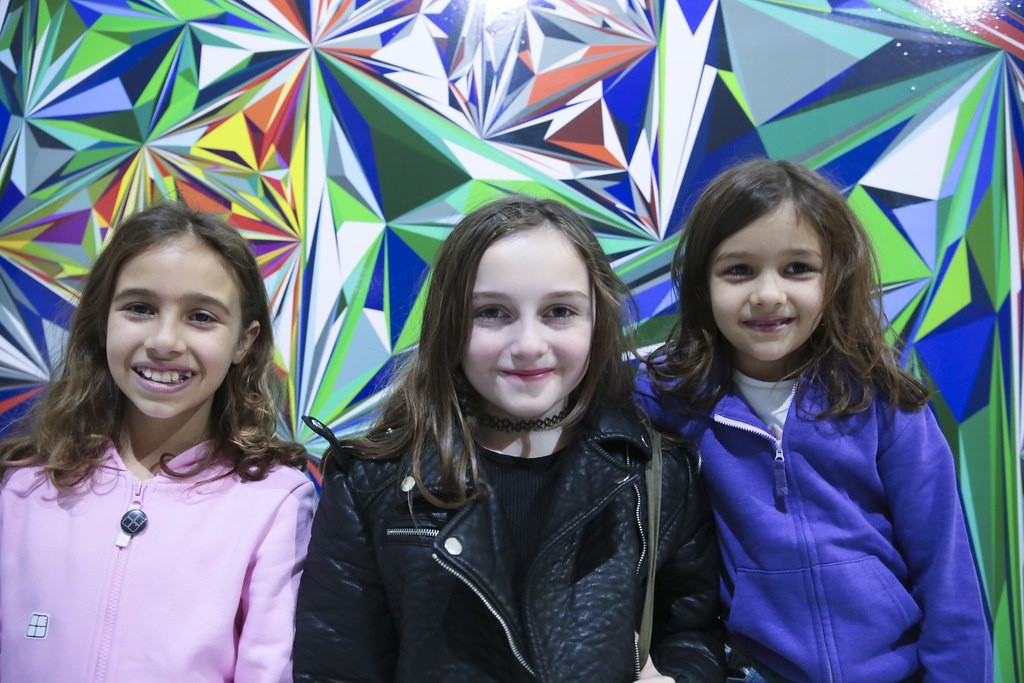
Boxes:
[628,159,992,683]
[0,204,318,682]
[293,193,726,683]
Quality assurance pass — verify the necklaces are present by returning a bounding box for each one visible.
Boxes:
[470,406,568,431]
